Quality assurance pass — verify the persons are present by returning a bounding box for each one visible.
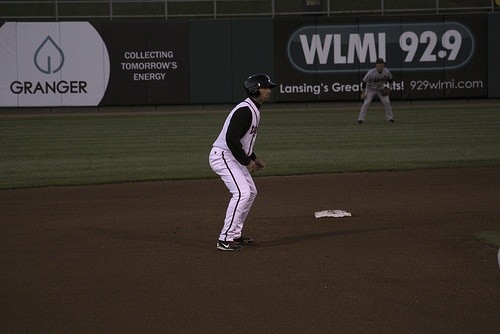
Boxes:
[209,74,276,251]
[357,58,395,125]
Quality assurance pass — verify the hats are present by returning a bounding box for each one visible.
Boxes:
[376,58,384,63]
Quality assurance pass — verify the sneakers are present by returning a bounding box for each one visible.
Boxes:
[217,240,241,250]
[233,236,252,244]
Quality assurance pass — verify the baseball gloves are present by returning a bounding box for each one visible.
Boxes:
[380,87,391,96]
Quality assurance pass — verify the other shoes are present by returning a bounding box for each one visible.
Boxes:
[358,121,362,123]
[389,120,393,123]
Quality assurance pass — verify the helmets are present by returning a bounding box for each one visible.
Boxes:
[244,74,277,98]
